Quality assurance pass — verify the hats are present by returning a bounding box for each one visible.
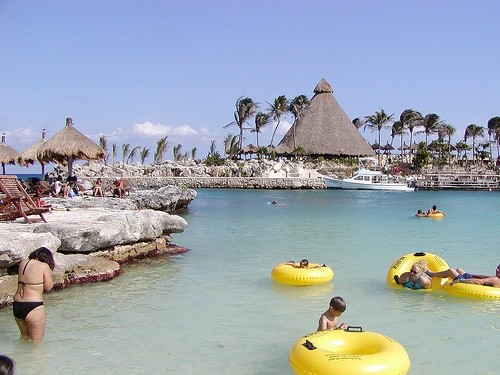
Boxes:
[115,177,120,179]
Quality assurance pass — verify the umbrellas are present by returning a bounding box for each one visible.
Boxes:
[0,132,21,174]
[18,129,67,180]
[226,144,294,160]
[37,117,107,177]
[371,140,456,154]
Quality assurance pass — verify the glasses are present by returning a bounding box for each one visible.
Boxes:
[410,269,419,274]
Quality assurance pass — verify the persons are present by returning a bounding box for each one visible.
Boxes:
[428,205,439,213]
[317,296,348,331]
[272,200,277,204]
[13,247,54,339]
[404,179,413,187]
[66,175,78,194]
[0,355,16,375]
[26,175,70,198]
[92,178,103,197]
[415,209,427,216]
[425,264,500,286]
[282,259,320,268]
[383,165,399,175]
[399,258,432,289]
[110,177,124,198]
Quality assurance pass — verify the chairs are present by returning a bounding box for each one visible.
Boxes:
[0,174,54,224]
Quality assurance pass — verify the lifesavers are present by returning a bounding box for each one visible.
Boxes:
[289,330,410,375]
[446,280,500,300]
[388,253,451,291]
[273,263,334,285]
[429,213,444,218]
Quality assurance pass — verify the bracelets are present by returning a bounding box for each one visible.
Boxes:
[460,278,464,282]
